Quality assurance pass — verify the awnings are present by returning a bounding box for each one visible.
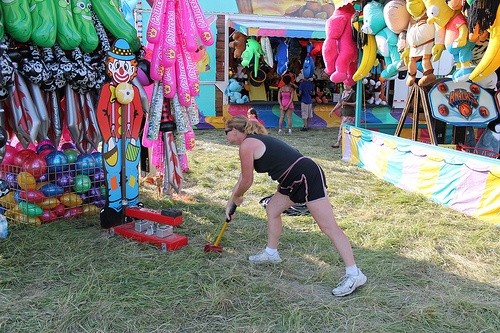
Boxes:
[230,17,327,39]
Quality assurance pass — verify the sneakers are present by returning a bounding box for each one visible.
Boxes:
[333,268,367,296]
[249,249,282,264]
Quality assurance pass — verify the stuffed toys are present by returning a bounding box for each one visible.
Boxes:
[323,0,500,85]
[224,30,393,106]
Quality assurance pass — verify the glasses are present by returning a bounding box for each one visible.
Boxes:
[225,129,232,135]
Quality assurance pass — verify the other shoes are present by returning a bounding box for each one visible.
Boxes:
[301,127,308,131]
[332,145,339,148]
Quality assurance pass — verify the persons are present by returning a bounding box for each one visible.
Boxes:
[225,115,368,298]
[296,73,316,133]
[240,108,268,143]
[277,75,295,137]
[329,77,357,150]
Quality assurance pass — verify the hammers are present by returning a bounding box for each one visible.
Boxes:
[204,203,237,253]
